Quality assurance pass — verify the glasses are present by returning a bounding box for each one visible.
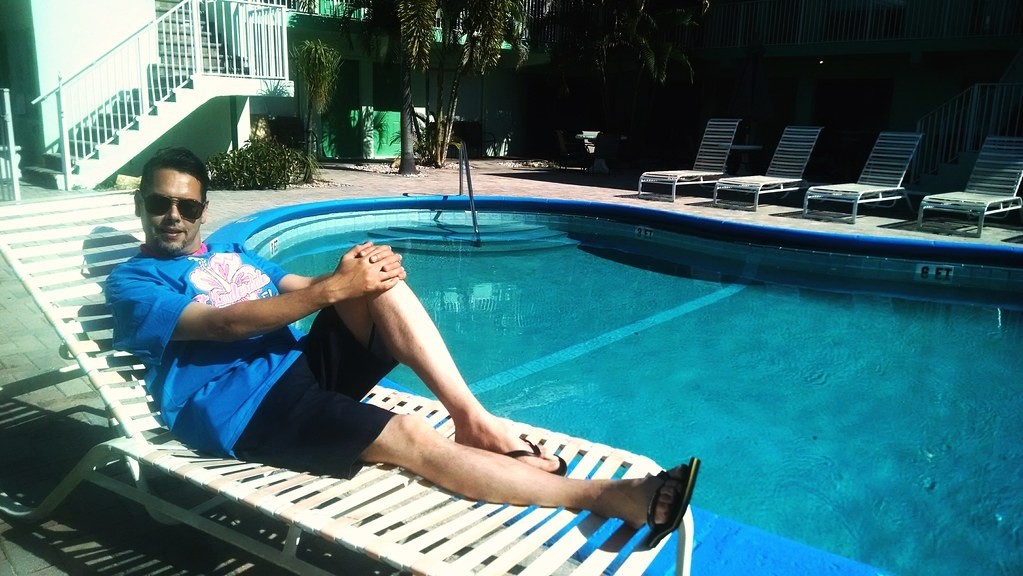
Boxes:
[142,192,208,221]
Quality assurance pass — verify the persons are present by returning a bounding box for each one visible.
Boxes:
[104,149,700,548]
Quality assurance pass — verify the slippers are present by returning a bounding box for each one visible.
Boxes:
[645,457,702,550]
[500,437,568,478]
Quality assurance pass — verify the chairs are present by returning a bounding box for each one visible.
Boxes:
[802,131,925,225]
[637,118,741,202]
[713,126,826,212]
[917,136,1023,238]
[0,189,694,576]
